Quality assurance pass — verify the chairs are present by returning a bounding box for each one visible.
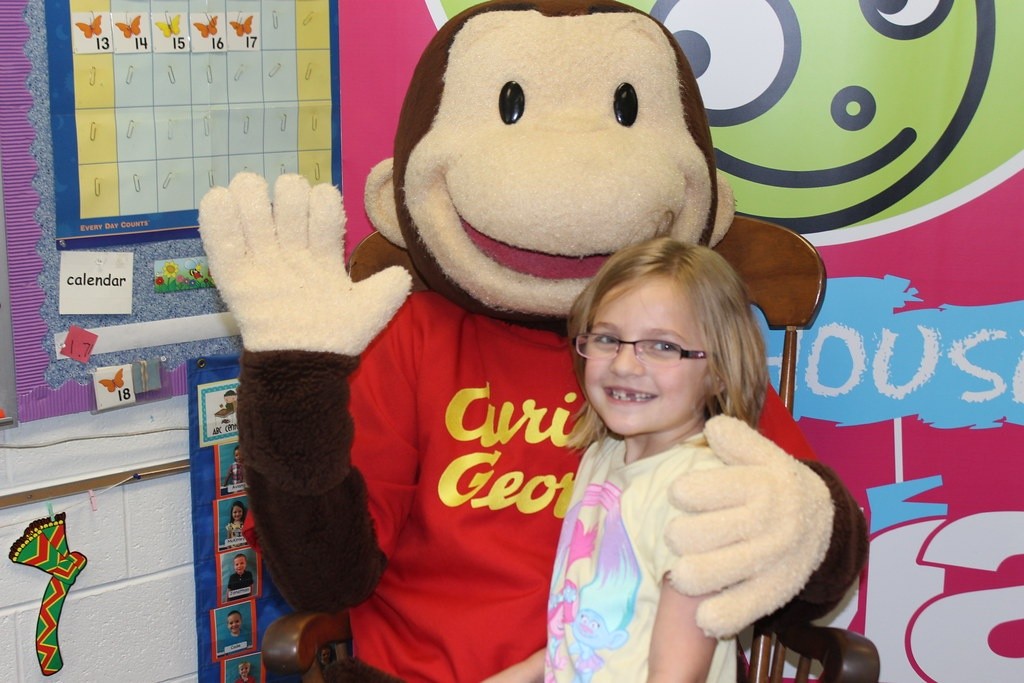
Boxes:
[262,213,880,683]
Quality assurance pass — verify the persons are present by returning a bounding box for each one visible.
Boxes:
[224,610,249,655]
[225,501,248,548]
[226,554,254,601]
[318,645,333,668]
[224,446,243,486]
[235,662,255,683]
[480,236,768,683]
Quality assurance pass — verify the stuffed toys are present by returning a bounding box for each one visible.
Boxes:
[198,1,870,683]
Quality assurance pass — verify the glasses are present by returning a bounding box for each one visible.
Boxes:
[577,334,707,366]
